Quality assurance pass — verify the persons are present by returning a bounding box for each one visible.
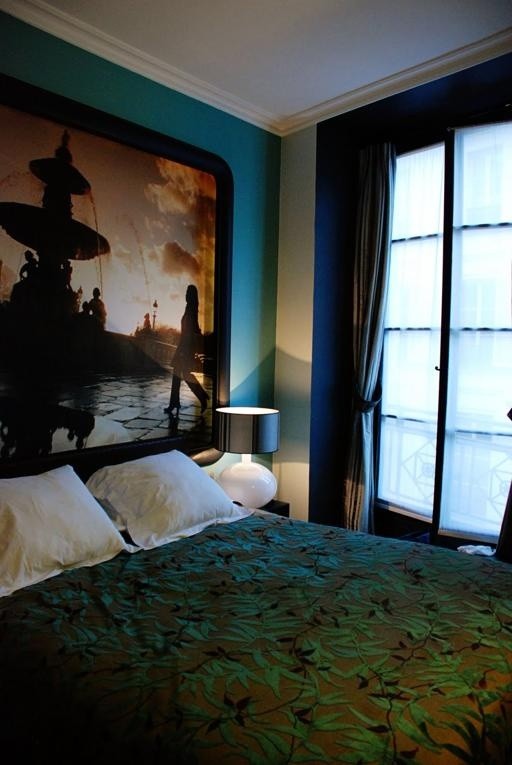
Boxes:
[11,250,108,323]
[142,313,154,327]
[162,284,209,414]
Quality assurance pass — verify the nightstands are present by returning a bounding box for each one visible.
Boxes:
[231,498,289,519]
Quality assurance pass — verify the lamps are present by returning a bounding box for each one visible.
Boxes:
[212,405,281,508]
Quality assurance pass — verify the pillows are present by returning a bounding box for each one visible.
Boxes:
[84,450,254,551]
[0,464,130,596]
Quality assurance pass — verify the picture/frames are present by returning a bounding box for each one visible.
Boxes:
[0,73,235,477]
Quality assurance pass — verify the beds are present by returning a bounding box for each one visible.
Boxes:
[0,505,511,764]
